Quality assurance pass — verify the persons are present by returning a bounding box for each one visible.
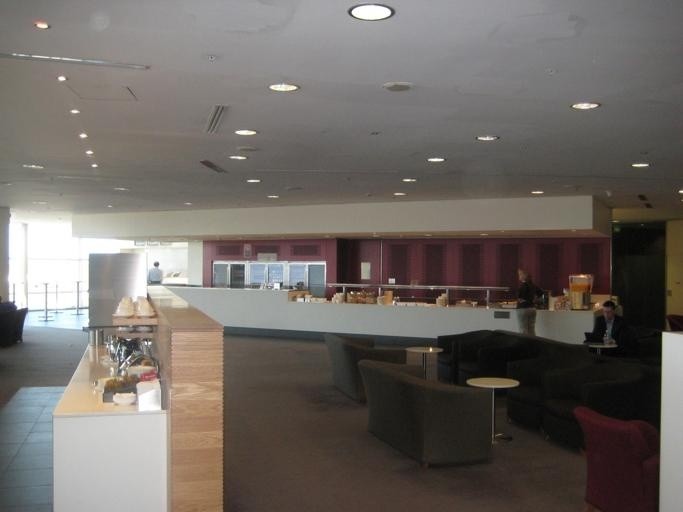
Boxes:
[592,300,629,346]
[518,269,543,309]
[147,261,162,284]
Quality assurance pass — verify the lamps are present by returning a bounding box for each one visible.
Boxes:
[201,104,229,136]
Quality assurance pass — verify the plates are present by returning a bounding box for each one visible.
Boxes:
[137,314,156,317]
[112,313,134,317]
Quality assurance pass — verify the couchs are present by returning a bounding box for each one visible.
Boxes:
[453,333,538,398]
[355,358,495,467]
[327,333,413,401]
[572,405,660,512]
[505,349,594,435]
[542,360,658,457]
[436,330,509,385]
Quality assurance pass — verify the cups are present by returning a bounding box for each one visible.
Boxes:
[88,329,104,346]
[116,296,155,313]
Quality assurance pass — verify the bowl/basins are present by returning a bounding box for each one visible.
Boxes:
[112,392,137,407]
